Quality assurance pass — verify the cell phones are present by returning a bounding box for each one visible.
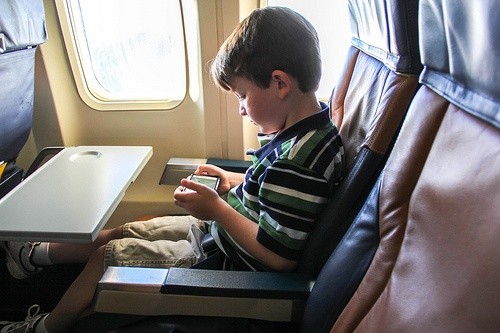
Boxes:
[183,174,221,193]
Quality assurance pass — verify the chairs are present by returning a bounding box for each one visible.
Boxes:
[90,0,500,333]
[0,0,61,200]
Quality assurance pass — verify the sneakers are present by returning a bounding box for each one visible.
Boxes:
[0,305,47,333]
[0,238,44,280]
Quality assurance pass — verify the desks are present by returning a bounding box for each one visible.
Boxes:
[0,145,154,243]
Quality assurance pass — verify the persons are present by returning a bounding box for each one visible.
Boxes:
[0,5,344,333]
